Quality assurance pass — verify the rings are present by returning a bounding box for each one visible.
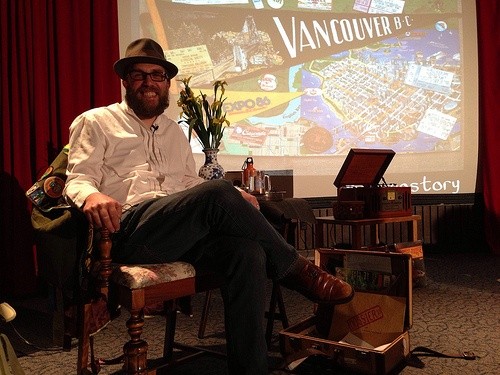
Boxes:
[256,204,259,207]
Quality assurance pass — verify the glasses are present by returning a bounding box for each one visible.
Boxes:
[126,69,168,82]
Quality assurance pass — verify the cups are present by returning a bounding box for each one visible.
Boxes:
[255,170,271,195]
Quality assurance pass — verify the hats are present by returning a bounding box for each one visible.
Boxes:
[113,38,178,80]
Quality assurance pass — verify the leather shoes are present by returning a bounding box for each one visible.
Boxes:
[278,253,355,305]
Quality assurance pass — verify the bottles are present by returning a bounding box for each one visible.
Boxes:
[241,157,255,193]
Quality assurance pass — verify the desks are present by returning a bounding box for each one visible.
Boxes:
[315,213,428,282]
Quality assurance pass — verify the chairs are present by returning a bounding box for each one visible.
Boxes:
[34,141,196,375]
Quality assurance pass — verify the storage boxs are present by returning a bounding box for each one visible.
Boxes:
[334,147,412,216]
[277,244,414,373]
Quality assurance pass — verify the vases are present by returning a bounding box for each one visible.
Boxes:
[199,147,226,182]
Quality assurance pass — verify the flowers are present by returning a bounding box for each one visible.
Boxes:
[176,76,233,149]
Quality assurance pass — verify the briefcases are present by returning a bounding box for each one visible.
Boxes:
[280,246,413,375]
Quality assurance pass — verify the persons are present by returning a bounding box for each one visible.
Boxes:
[61,39,354,375]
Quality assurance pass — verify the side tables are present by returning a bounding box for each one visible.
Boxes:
[249,197,317,346]
